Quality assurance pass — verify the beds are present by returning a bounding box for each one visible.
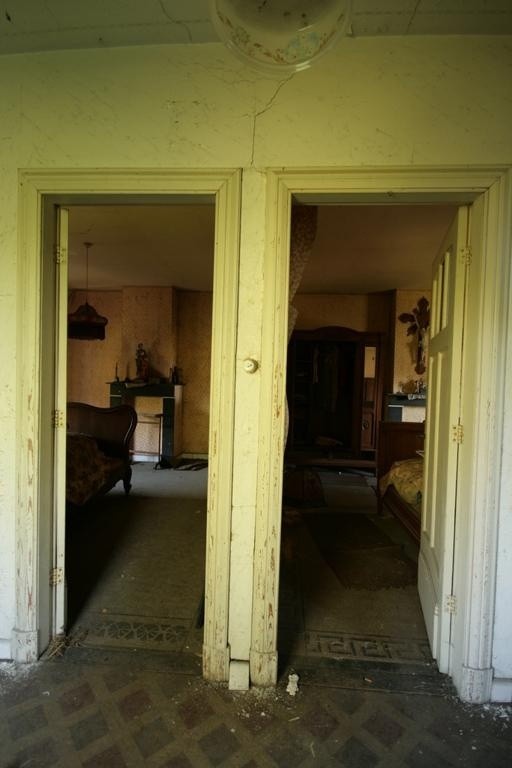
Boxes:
[66,401,138,512]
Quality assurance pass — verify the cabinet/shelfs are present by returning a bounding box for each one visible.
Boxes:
[286,325,385,468]
[106,381,183,468]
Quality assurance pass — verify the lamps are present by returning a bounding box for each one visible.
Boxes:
[67,242,108,342]
[210,0,356,76]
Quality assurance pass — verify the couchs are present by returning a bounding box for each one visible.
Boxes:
[377,420,426,548]
